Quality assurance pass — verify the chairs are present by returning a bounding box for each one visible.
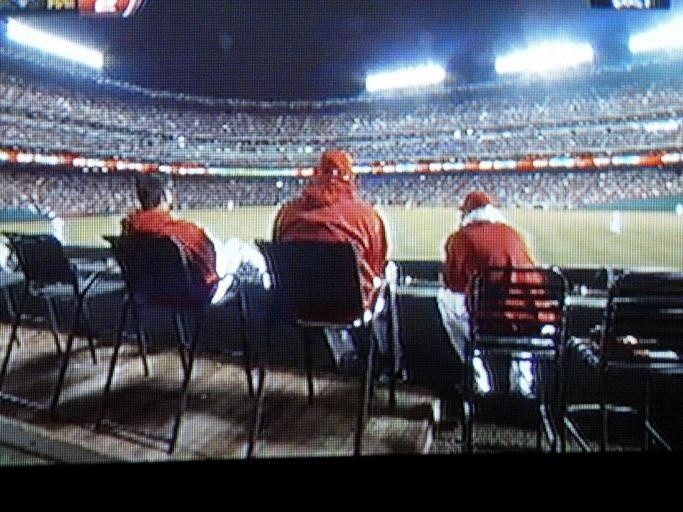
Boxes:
[564,273,683,452]
[246,239,395,457]
[0,231,149,410]
[95,234,255,454]
[461,266,564,453]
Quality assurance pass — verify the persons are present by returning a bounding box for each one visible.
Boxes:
[272,149,390,387]
[435,190,559,399]
[120,170,247,357]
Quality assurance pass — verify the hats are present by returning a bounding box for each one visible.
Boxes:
[459,192,489,209]
[320,151,350,178]
